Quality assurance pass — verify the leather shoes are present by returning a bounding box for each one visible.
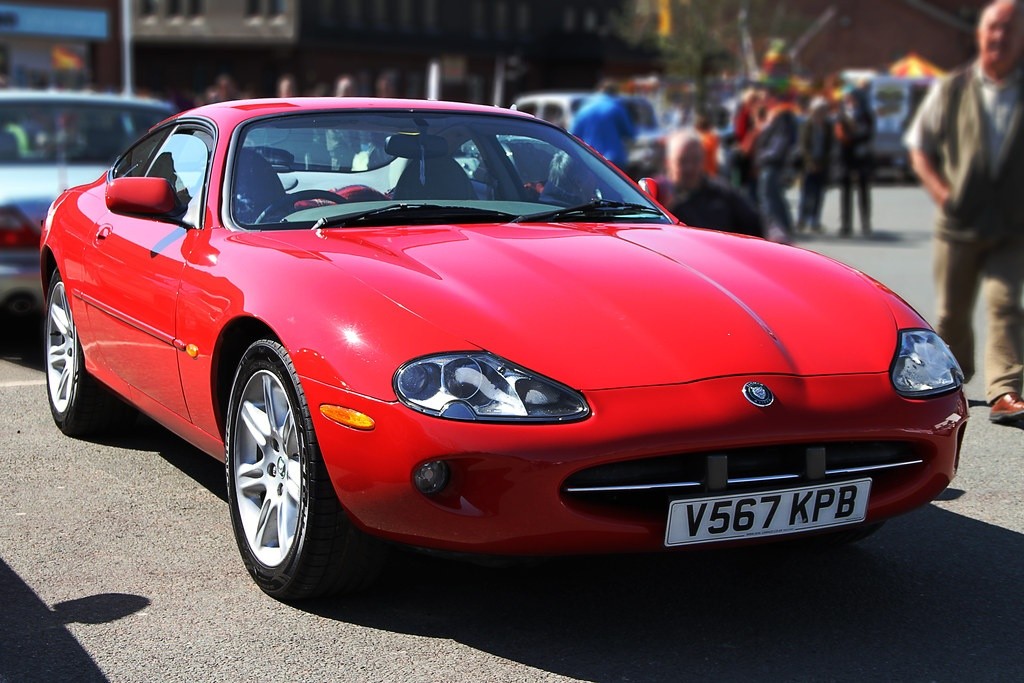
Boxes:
[989,392,1024,423]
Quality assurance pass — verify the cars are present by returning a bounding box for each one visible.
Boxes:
[37,97,970,606]
[0,86,180,316]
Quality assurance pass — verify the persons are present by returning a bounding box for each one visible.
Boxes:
[624,51,913,241]
[902,1,1023,425]
[146,151,191,223]
[0,63,540,230]
[540,153,599,210]
[569,77,636,175]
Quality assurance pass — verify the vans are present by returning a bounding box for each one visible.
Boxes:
[461,93,663,181]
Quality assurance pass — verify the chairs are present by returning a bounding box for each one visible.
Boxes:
[198,150,294,224]
[392,155,479,202]
